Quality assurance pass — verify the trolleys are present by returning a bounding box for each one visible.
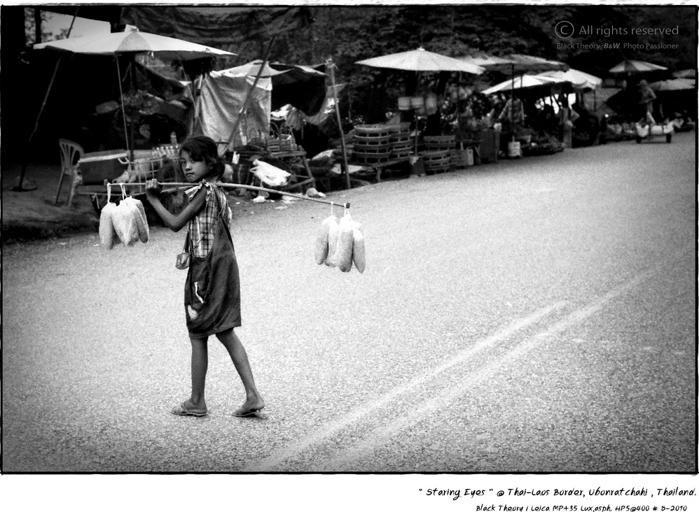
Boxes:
[635,100,672,143]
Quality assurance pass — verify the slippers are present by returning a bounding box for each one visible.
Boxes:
[171,402,206,417]
[231,405,270,417]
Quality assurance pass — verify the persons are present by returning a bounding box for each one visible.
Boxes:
[144,136,267,419]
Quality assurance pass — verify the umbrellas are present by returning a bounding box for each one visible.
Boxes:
[354,45,486,158]
[455,50,697,143]
[32,24,242,149]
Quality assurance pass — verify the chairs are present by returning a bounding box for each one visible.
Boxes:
[53,139,85,211]
